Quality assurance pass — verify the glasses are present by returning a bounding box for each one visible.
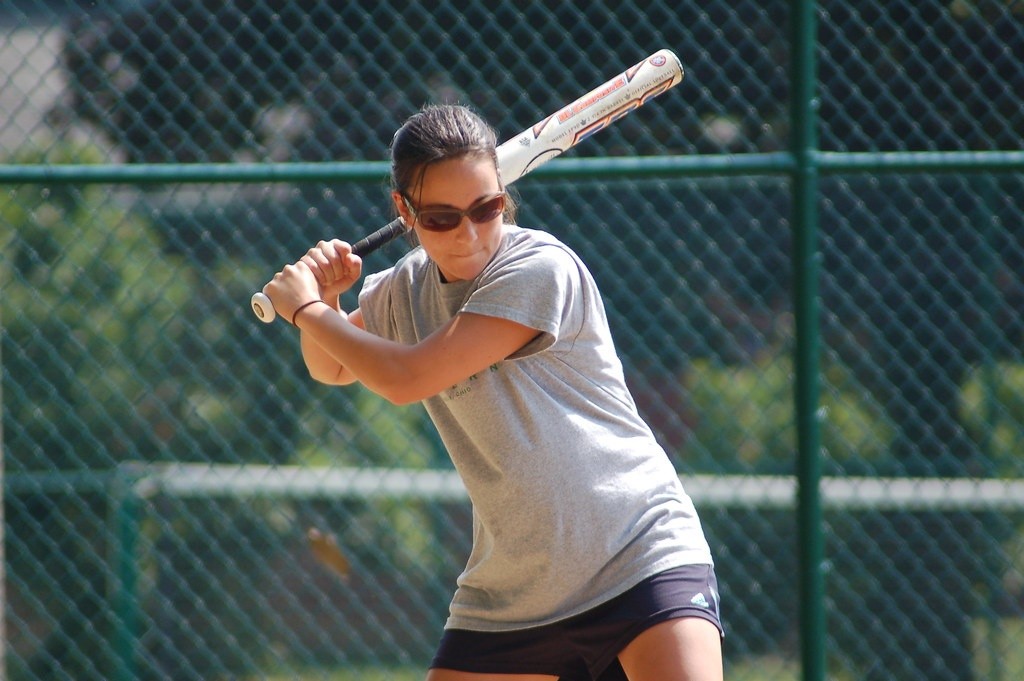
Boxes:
[402,192,504,232]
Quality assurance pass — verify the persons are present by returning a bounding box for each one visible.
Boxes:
[261,104,726,681]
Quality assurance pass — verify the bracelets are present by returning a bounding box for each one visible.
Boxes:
[292,299,325,327]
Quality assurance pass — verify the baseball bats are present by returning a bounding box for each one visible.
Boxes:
[250,48,687,324]
